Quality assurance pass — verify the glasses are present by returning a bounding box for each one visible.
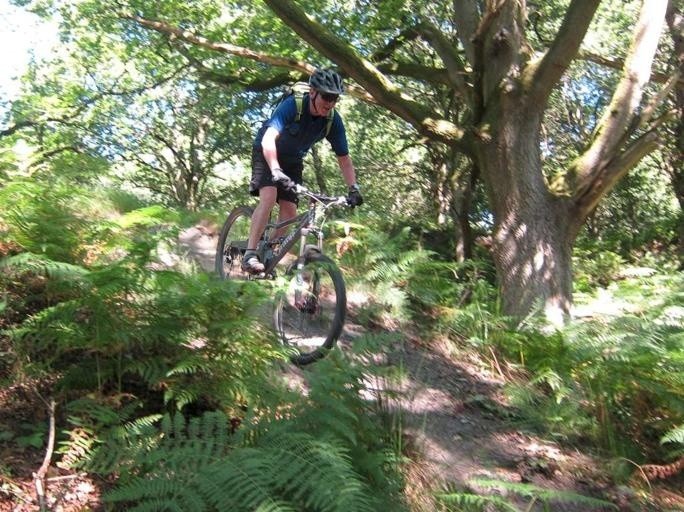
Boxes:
[319,92,339,102]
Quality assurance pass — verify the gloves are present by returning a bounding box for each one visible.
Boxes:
[271,168,291,189]
[346,183,364,209]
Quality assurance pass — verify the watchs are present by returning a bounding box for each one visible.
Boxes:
[347,182,359,192]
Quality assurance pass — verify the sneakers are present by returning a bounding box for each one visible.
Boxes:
[240,256,265,274]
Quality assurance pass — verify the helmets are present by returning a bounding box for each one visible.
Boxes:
[309,68,345,95]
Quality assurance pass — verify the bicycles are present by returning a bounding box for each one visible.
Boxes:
[215,176,364,365]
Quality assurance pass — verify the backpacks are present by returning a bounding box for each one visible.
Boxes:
[273,81,335,139]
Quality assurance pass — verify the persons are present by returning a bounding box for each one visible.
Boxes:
[239,68,364,283]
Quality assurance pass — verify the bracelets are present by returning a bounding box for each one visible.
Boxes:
[269,167,283,175]
